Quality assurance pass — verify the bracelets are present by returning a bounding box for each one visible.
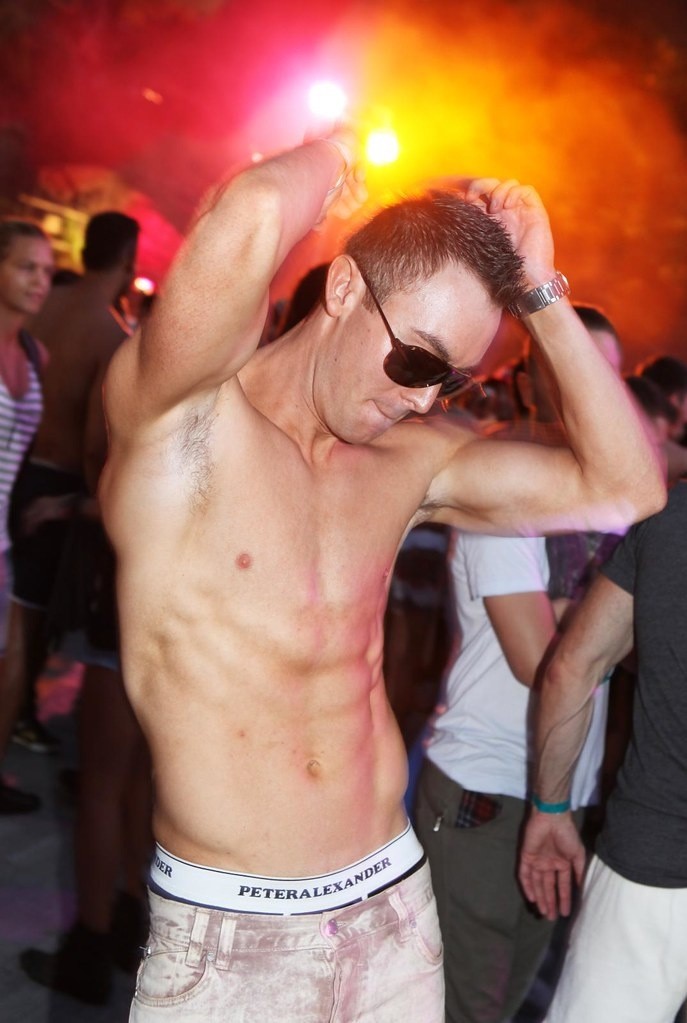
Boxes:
[504,271,570,323]
[317,137,351,195]
[532,793,572,814]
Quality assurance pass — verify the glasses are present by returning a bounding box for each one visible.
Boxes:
[353,256,471,398]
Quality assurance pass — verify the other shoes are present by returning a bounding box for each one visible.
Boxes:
[66,895,143,970]
[21,924,114,1004]
[0,786,40,814]
[9,716,55,753]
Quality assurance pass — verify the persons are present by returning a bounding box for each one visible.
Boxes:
[406,301,623,1023]
[515,484,687,1023]
[17,639,156,1008]
[0,210,687,822]
[94,121,668,1023]
